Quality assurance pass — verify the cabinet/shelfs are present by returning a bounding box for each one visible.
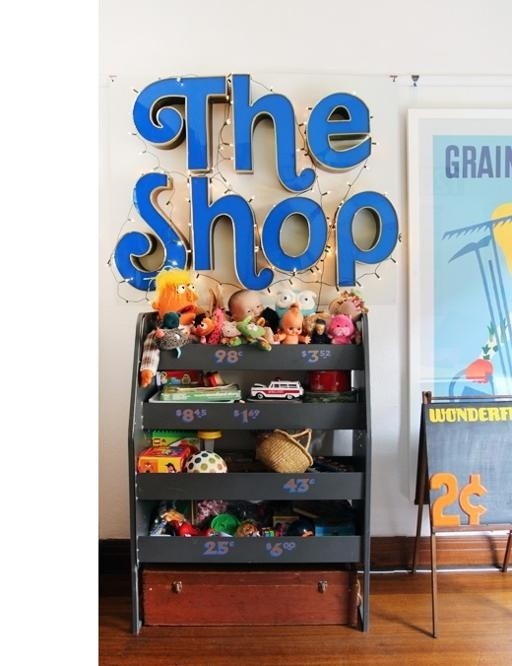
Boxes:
[126,307,372,636]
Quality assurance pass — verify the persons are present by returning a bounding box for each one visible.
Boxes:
[139,263,369,389]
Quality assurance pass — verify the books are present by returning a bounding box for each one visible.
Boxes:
[159,384,243,402]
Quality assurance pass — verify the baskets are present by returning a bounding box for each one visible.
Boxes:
[255,426,317,474]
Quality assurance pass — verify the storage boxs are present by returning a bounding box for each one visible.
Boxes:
[141,568,361,629]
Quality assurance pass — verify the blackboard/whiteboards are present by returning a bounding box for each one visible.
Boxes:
[423,402,512,532]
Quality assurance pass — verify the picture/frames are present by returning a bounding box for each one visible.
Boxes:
[406,109,512,502]
